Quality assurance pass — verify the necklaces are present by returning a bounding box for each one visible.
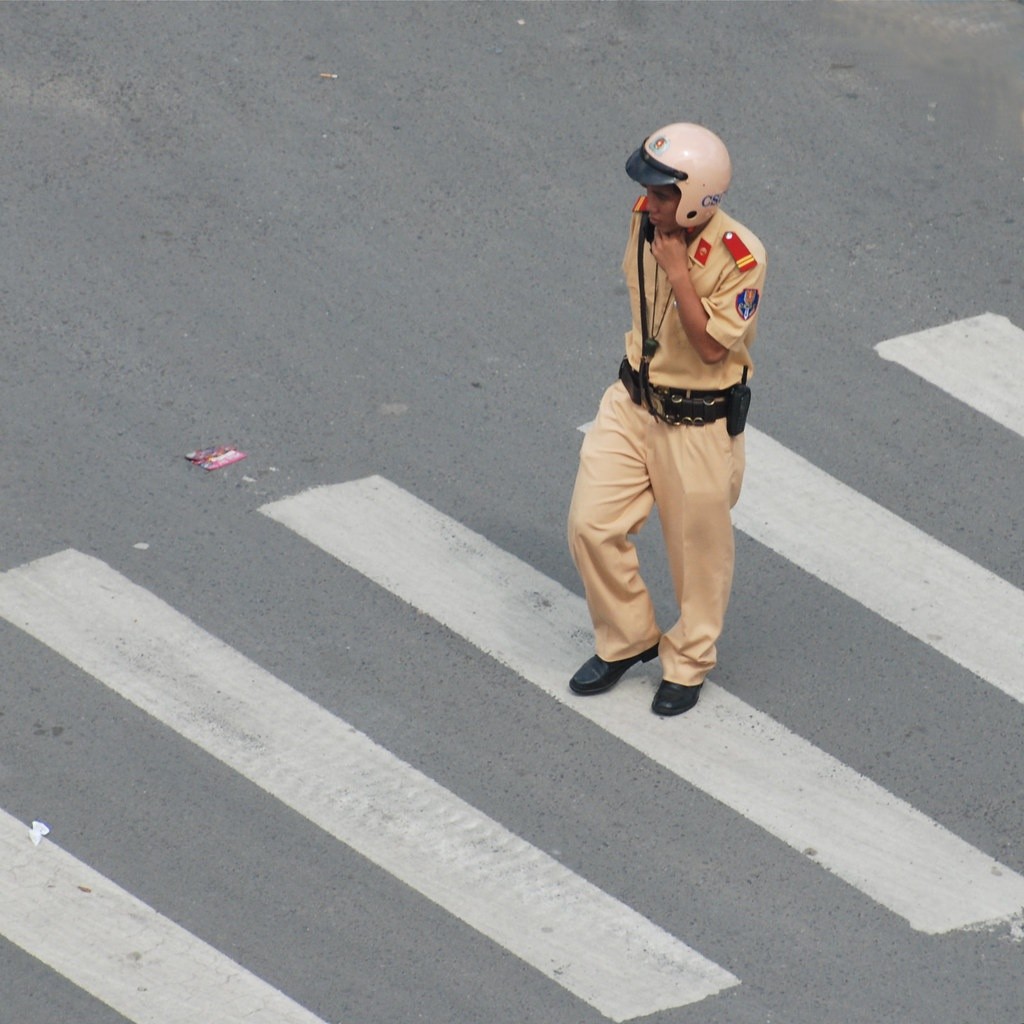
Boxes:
[645,260,673,357]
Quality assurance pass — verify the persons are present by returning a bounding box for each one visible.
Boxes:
[567,123,767,717]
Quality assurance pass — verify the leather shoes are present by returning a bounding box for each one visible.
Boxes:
[569,642,659,696]
[651,679,704,717]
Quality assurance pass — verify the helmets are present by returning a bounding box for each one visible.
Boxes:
[625,121,733,228]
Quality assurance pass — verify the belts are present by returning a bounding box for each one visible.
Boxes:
[618,358,728,426]
